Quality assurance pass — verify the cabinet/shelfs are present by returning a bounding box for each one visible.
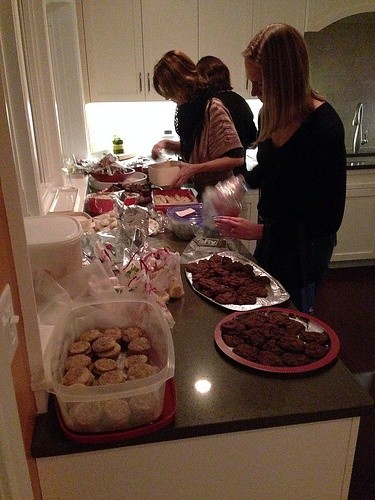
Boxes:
[81,1,306,102]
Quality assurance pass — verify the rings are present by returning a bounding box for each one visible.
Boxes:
[231,228,234,232]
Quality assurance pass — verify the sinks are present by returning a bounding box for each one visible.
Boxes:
[346,156,375,166]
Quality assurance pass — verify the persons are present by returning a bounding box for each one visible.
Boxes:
[214,24,348,316]
[196,56,257,177]
[153,49,244,204]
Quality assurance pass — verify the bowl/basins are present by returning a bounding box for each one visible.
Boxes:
[147,161,181,187]
[167,203,208,241]
[42,294,176,433]
[87,191,140,216]
[90,168,135,183]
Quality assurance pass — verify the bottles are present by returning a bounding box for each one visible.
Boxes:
[162,130,176,159]
[112,137,124,154]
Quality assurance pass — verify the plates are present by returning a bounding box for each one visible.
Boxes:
[214,306,340,374]
[94,212,160,238]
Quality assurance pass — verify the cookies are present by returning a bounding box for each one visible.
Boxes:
[63,325,162,431]
[185,254,270,304]
[220,312,330,368]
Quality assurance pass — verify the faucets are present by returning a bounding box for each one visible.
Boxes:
[351,102,368,154]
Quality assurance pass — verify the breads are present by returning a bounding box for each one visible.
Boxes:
[105,182,151,197]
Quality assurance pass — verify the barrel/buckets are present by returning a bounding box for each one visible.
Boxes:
[24,214,83,289]
